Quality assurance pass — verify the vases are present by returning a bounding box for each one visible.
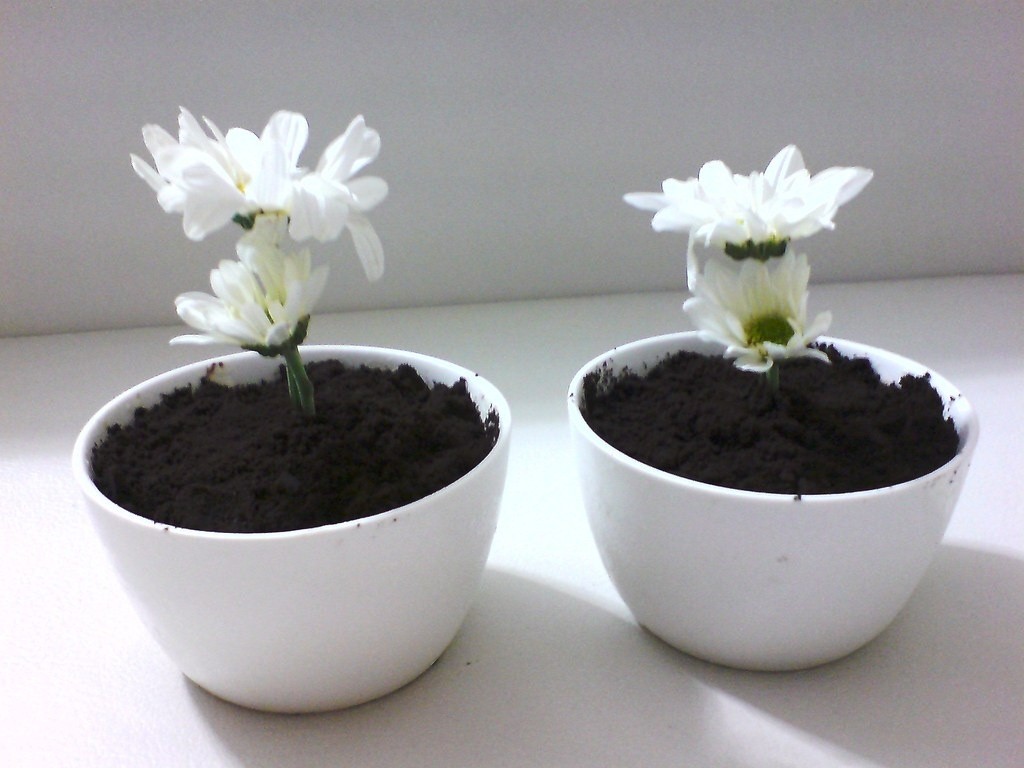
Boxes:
[561,329,983,672]
[72,344,519,717]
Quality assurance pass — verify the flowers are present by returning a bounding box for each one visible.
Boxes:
[623,145,874,390]
[127,104,393,412]
